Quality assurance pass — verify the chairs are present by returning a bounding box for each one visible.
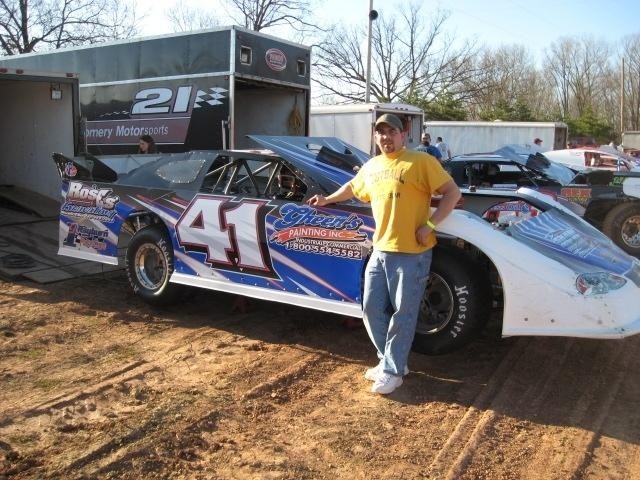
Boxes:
[479,164,500,185]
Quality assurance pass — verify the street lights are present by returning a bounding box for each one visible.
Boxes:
[365,0,378,104]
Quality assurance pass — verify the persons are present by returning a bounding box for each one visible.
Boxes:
[531,138,543,153]
[306,113,462,395]
[412,134,443,165]
[137,135,162,154]
[435,137,451,161]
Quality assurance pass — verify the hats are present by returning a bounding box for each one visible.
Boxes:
[374,113,403,132]
[533,138,543,144]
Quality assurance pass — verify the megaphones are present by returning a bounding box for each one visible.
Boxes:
[369,10,378,20]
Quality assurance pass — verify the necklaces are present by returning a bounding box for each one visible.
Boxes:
[427,220,436,229]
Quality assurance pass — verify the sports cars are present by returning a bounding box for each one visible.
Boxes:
[50,146,639,356]
[242,135,640,260]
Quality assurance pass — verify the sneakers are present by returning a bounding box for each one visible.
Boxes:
[372,371,403,394]
[364,358,410,381]
